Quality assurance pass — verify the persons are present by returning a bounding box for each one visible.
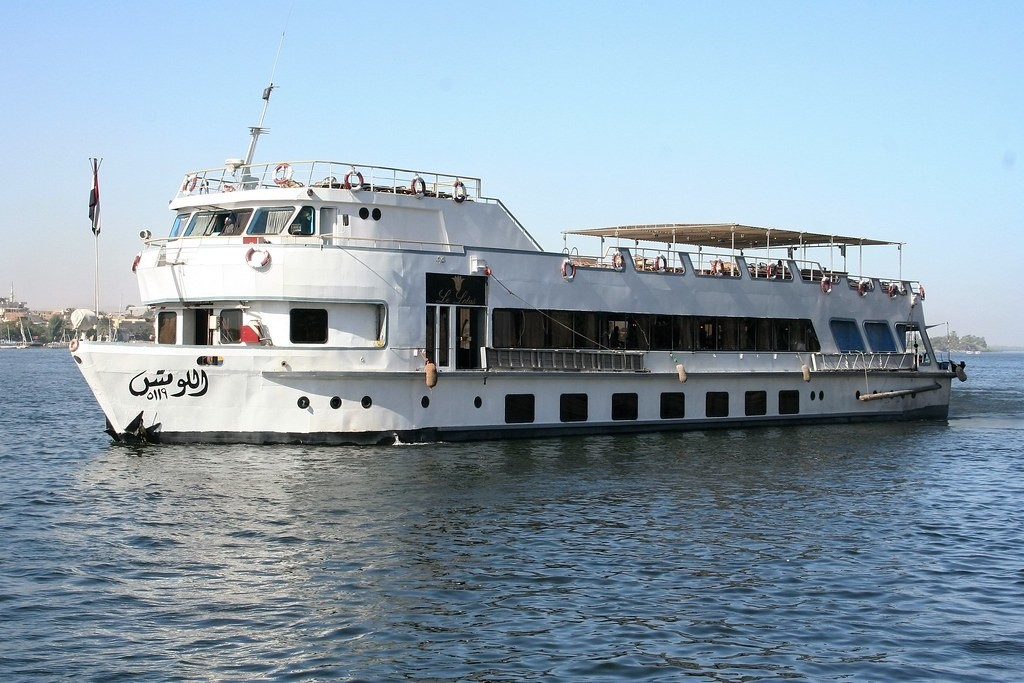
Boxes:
[610,326,620,349]
[222,216,236,234]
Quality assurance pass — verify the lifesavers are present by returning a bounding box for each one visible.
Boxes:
[767,261,779,280]
[68,338,79,352]
[451,180,466,204]
[272,163,294,184]
[182,176,197,195]
[561,259,578,281]
[653,253,667,273]
[613,252,625,272]
[199,184,210,194]
[888,284,898,299]
[920,288,925,300]
[821,276,833,294]
[411,176,426,198]
[245,245,271,267]
[132,254,140,271]
[345,168,364,192]
[712,257,725,276]
[858,281,870,298]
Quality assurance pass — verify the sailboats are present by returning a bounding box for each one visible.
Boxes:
[0,313,43,348]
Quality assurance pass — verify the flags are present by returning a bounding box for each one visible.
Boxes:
[87,160,102,237]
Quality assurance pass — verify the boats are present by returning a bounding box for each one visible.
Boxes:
[70,6,967,442]
[48,329,70,348]
